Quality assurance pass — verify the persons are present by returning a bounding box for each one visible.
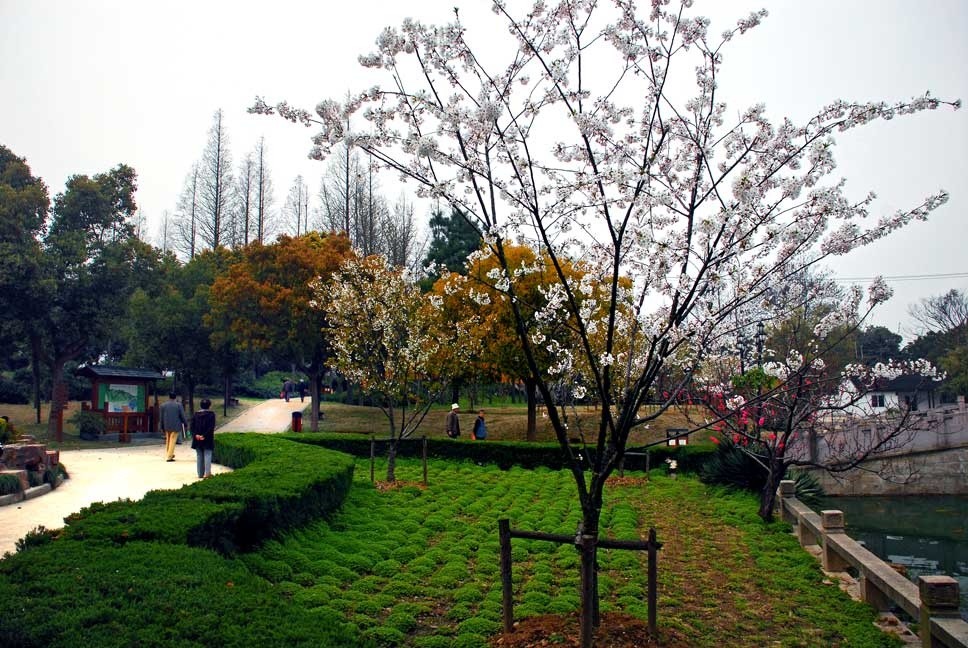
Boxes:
[0,416,8,438]
[473,410,486,440]
[446,403,460,439]
[189,399,216,478]
[282,378,291,402]
[158,391,188,462]
[297,379,305,402]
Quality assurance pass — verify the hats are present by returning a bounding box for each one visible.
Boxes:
[452,403,459,410]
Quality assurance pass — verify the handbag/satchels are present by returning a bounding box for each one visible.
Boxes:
[280,391,285,398]
[304,389,307,392]
[471,432,475,440]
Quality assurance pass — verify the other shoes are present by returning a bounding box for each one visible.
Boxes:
[203,473,212,478]
[301,398,304,402]
[167,459,175,461]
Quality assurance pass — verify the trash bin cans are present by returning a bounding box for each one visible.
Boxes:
[230,399,239,405]
[292,412,303,433]
[666,429,689,446]
